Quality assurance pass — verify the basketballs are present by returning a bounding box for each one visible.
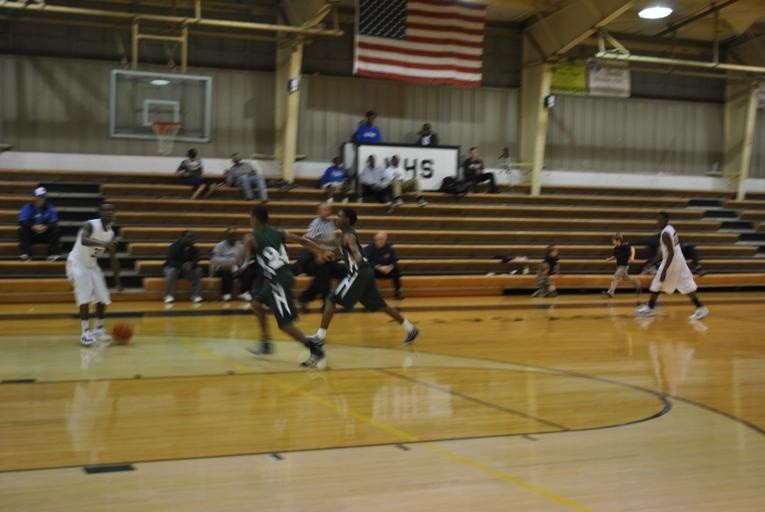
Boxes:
[111,322,134,346]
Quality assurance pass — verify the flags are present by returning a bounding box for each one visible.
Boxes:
[353,0,487,88]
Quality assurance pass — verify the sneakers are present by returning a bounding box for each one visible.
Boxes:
[47,254,61,261]
[18,254,32,262]
[603,291,613,298]
[93,330,112,341]
[633,302,657,317]
[162,290,418,368]
[326,196,429,214]
[79,334,96,345]
[689,305,711,320]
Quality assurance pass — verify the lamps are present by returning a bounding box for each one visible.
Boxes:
[288,77,299,93]
[545,92,555,108]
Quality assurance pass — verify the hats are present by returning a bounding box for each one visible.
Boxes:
[32,187,47,197]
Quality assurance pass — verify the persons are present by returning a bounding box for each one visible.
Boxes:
[602,231,644,297]
[174,149,218,199]
[316,109,503,209]
[163,230,404,309]
[17,187,65,262]
[249,203,419,367]
[528,245,564,320]
[224,151,269,203]
[65,202,124,345]
[635,211,710,321]
